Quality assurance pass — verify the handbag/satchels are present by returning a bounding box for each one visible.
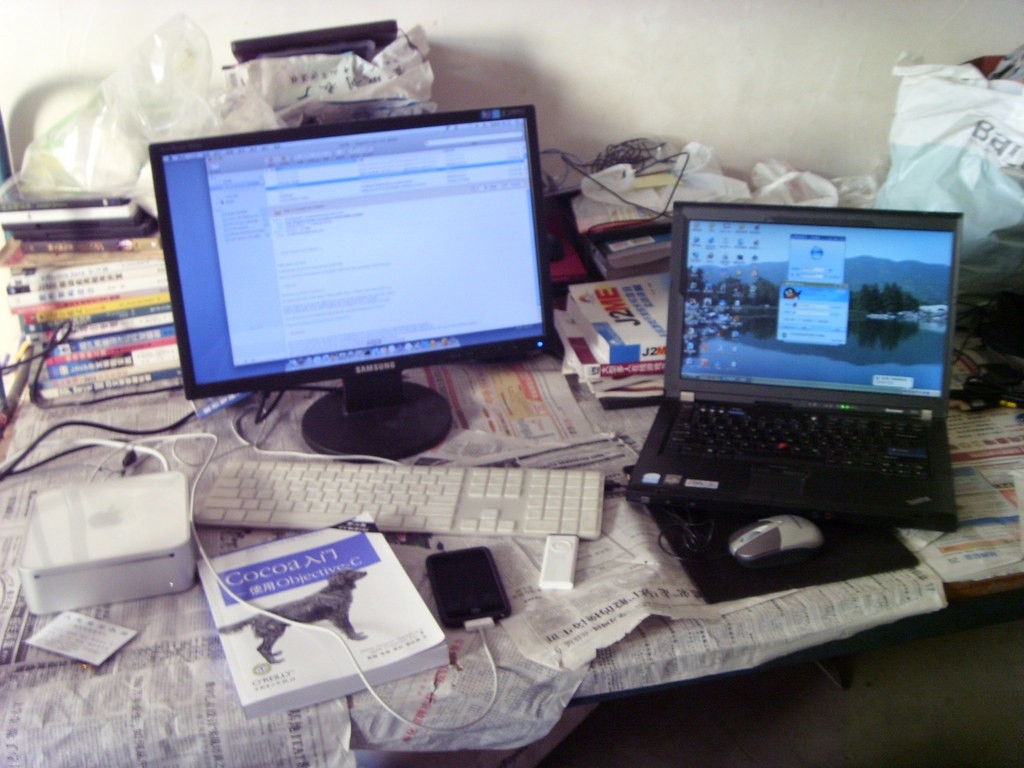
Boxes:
[866,52,1024,295]
[18,15,288,220]
[952,290,1024,362]
[215,25,438,135]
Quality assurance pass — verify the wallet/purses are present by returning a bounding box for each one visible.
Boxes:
[229,18,397,62]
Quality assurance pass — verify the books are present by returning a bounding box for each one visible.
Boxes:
[0,182,181,403]
[555,271,670,401]
[568,170,688,282]
[195,510,451,722]
[192,390,251,421]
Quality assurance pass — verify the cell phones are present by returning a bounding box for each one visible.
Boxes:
[425,545,512,631]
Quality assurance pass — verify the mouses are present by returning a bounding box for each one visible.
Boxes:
[729,514,823,567]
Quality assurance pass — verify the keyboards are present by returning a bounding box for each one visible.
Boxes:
[196,462,605,541]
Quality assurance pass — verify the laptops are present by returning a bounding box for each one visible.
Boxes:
[625,202,965,531]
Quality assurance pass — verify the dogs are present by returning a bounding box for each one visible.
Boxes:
[217,570,367,665]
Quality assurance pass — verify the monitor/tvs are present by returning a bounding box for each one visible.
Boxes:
[147,104,556,466]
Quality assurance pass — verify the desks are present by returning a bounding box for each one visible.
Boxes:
[0,358,1024,768]
